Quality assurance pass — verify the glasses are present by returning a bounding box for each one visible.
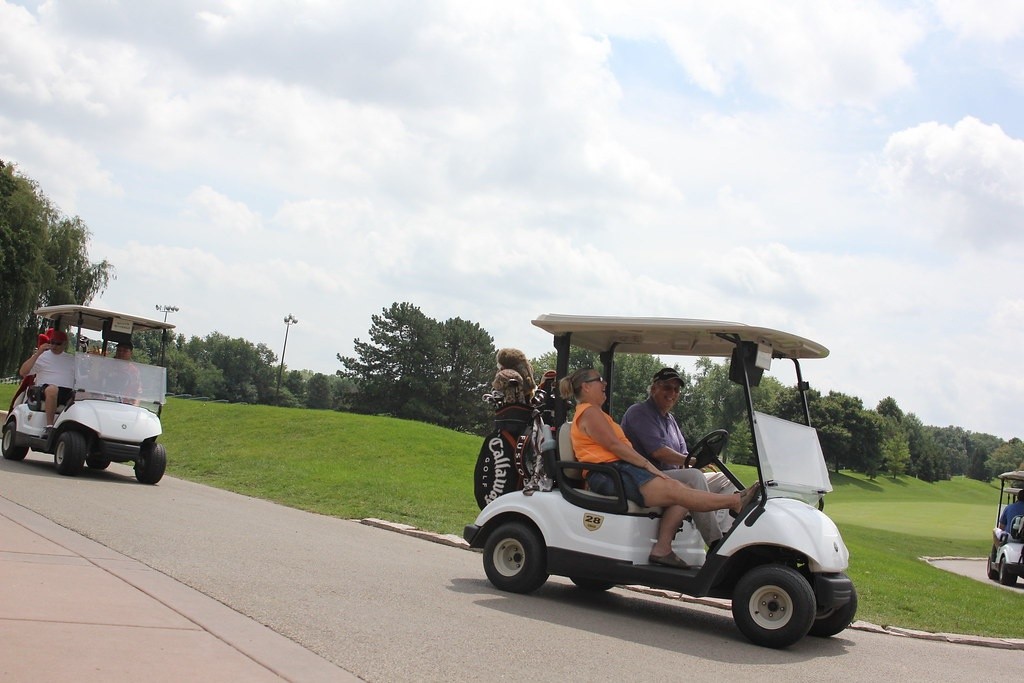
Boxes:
[49,340,63,346]
[657,383,681,393]
[584,377,604,383]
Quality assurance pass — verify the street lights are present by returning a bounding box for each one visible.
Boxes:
[270,313,298,407]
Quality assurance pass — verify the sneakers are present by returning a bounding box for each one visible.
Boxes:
[41,424,52,438]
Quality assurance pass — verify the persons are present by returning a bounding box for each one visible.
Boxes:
[620,367,745,549]
[559,366,762,571]
[19,331,85,440]
[991,490,1024,551]
[100,339,142,406]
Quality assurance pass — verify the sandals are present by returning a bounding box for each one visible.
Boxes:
[728,480,761,517]
[647,549,688,568]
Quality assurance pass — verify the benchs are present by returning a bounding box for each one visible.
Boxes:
[552,420,695,521]
[998,515,1024,548]
[28,383,66,413]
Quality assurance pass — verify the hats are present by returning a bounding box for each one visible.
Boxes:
[115,340,133,349]
[652,367,686,388]
[50,330,67,342]
